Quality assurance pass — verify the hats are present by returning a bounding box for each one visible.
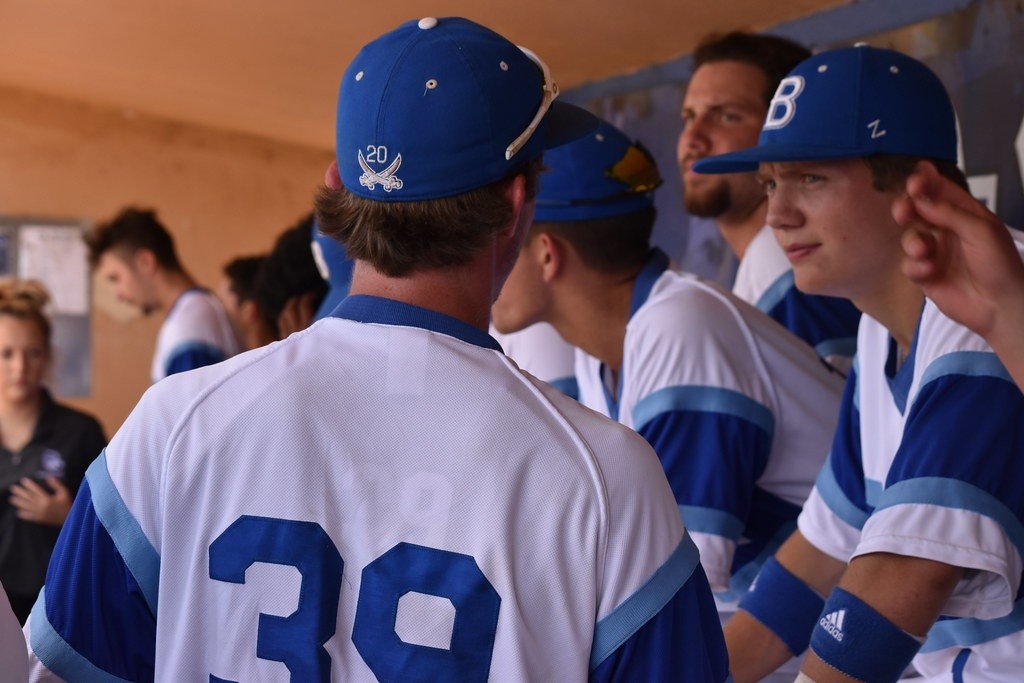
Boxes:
[690,42,959,174]
[310,218,355,325]
[335,17,558,198]
[524,100,664,222]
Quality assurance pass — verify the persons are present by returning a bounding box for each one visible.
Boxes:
[719,41,1024,682]
[220,204,355,354]
[33,13,735,682]
[488,308,583,404]
[486,99,850,624]
[84,203,242,384]
[893,155,1024,395]
[1,275,116,629]
[679,28,861,372]
